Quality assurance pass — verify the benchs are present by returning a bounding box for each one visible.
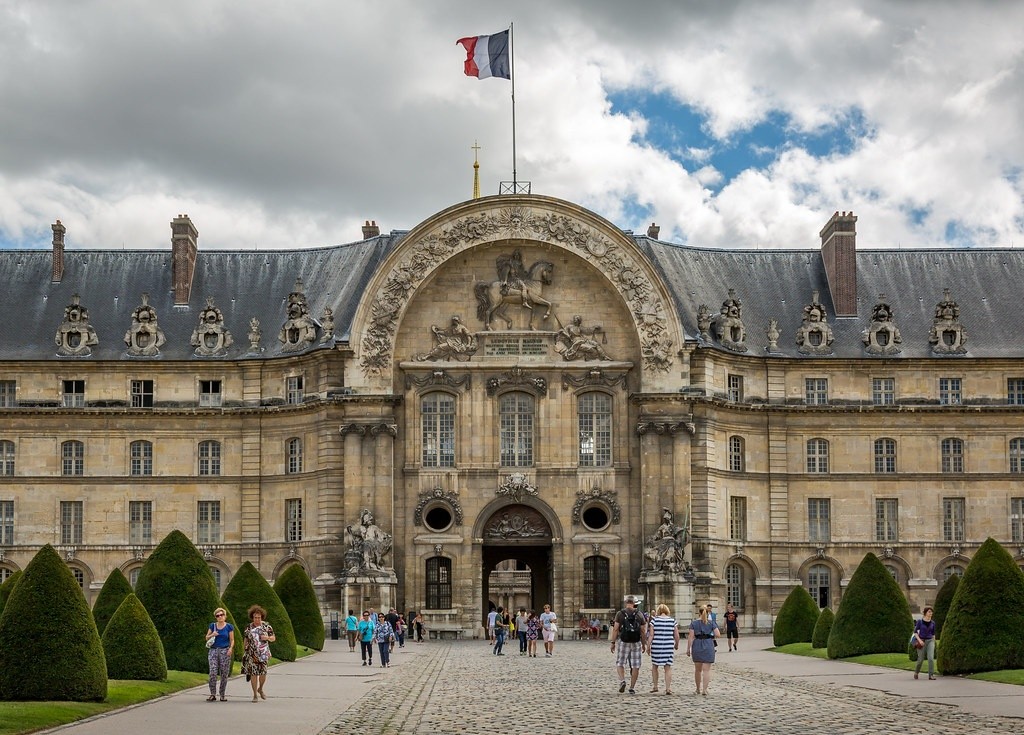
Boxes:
[573,628,608,641]
[428,627,467,640]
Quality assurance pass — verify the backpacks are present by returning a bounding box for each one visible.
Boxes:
[621,609,640,643]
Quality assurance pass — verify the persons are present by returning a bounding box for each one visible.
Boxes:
[205,607,235,702]
[241,605,275,702]
[344,607,425,668]
[914,607,937,680]
[486,604,557,657]
[502,249,533,310]
[419,316,473,361]
[644,507,686,568]
[563,316,612,361]
[580,595,739,695]
[346,511,392,574]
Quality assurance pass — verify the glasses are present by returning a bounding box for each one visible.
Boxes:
[215,613,225,618]
[363,614,370,617]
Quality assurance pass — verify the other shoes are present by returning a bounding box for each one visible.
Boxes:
[695,688,709,698]
[206,693,228,702]
[618,680,637,695]
[349,650,355,652]
[649,687,675,695]
[388,645,405,655]
[417,639,424,643]
[252,687,267,702]
[581,637,601,640]
[489,637,519,645]
[913,673,918,679]
[728,645,737,652]
[929,674,936,680]
[362,660,391,668]
[490,650,553,658]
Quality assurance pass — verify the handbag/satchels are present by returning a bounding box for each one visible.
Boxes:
[258,641,272,661]
[509,621,515,632]
[914,638,925,649]
[205,622,216,649]
[421,628,426,635]
[712,640,718,647]
[550,621,558,631]
[358,632,362,640]
[384,622,396,643]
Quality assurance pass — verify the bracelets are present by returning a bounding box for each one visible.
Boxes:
[611,640,616,644]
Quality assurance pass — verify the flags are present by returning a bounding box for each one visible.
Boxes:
[455,27,511,80]
